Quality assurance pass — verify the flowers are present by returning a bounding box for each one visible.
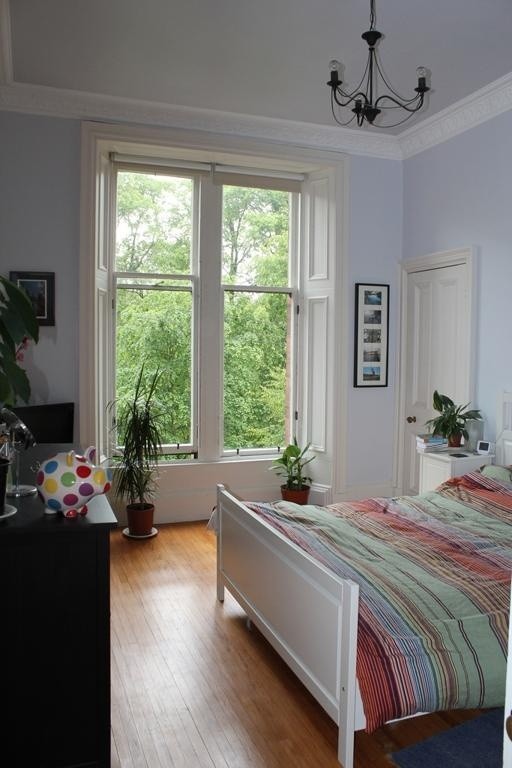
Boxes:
[0,275,40,409]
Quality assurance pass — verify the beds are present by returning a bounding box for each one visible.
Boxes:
[216,466,512,768]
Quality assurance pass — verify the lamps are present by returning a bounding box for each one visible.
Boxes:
[327,0,430,127]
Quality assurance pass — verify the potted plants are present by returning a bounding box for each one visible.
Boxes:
[422,391,482,447]
[267,434,318,505]
[101,363,176,536]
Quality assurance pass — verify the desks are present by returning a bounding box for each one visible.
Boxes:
[0,443,118,768]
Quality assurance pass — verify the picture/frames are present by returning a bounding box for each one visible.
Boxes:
[355,283,390,388]
[8,271,55,326]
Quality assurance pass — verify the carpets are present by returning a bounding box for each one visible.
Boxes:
[386,704,504,768]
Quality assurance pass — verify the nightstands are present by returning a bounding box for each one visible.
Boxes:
[418,451,495,497]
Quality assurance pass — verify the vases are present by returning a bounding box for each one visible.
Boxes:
[0,405,20,489]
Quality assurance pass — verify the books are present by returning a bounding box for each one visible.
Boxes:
[416,433,449,453]
